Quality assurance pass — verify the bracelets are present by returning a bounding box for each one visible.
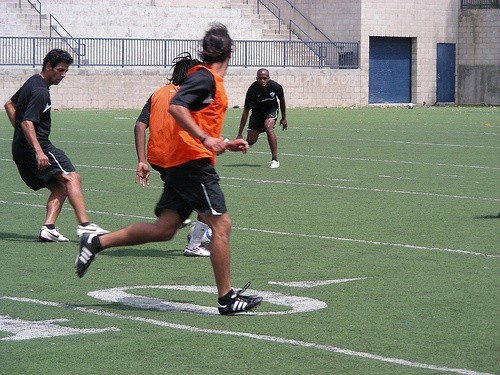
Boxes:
[198,133,208,142]
[224,138,229,144]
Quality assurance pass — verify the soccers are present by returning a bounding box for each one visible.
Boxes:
[187,223,213,249]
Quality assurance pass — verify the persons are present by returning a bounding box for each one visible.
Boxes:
[75,20,263,315]
[3,49,110,242]
[235,68,289,169]
[134,50,214,257]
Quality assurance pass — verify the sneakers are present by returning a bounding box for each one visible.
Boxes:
[75,233,101,278]
[183,244,210,257]
[39,226,69,241]
[217,281,263,315]
[76,223,112,239]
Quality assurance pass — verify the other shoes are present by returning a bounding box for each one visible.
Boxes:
[269,159,279,168]
